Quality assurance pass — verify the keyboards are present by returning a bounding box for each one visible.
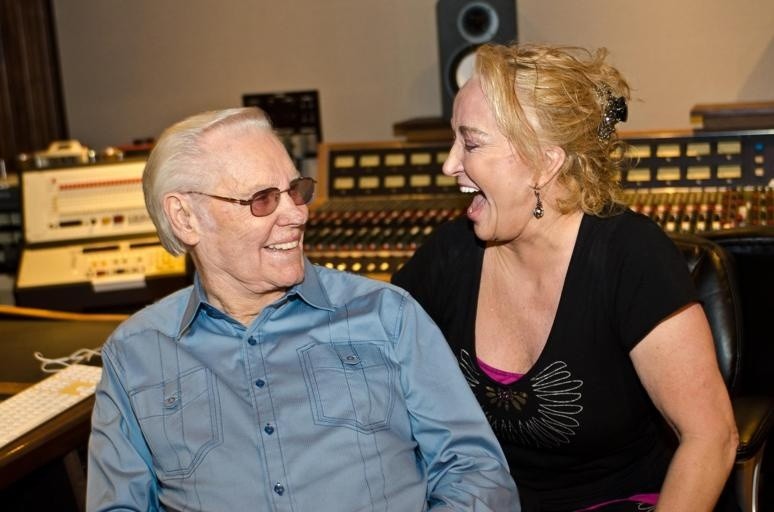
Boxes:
[0,363,103,449]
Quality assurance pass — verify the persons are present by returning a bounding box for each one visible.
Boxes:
[82,106,523,510]
[386,42,742,510]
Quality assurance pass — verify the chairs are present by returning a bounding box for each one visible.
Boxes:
[666,233,773,511]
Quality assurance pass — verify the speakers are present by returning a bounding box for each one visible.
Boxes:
[435,0,519,120]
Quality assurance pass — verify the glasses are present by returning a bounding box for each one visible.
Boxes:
[180,177,317,217]
